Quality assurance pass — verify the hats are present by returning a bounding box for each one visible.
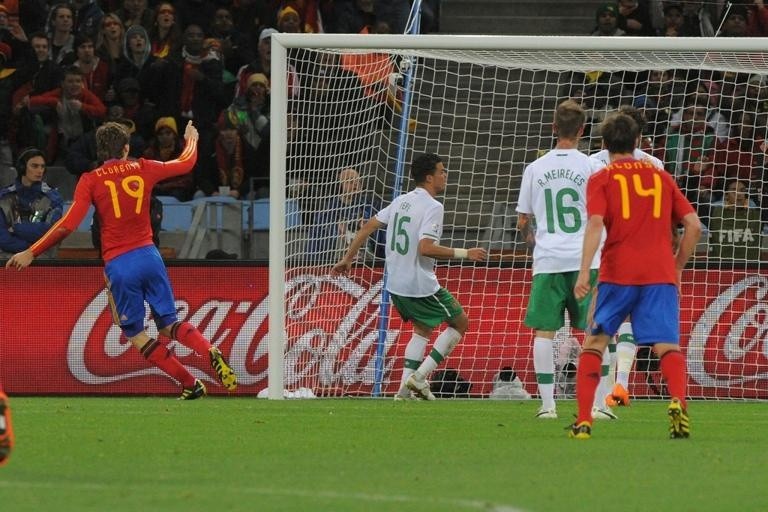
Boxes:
[259,28,279,46]
[247,73,269,90]
[156,117,178,135]
[277,6,300,29]
[207,249,237,259]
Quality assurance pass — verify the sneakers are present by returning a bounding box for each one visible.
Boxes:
[667,398,689,439]
[568,422,592,439]
[535,408,557,419]
[591,383,630,420]
[0,395,14,464]
[209,348,238,390]
[177,380,206,400]
[395,374,436,401]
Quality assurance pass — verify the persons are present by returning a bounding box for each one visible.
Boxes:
[587,103,668,407]
[1,0,768,269]
[565,113,705,440]
[329,152,488,403]
[4,119,239,402]
[515,98,619,421]
